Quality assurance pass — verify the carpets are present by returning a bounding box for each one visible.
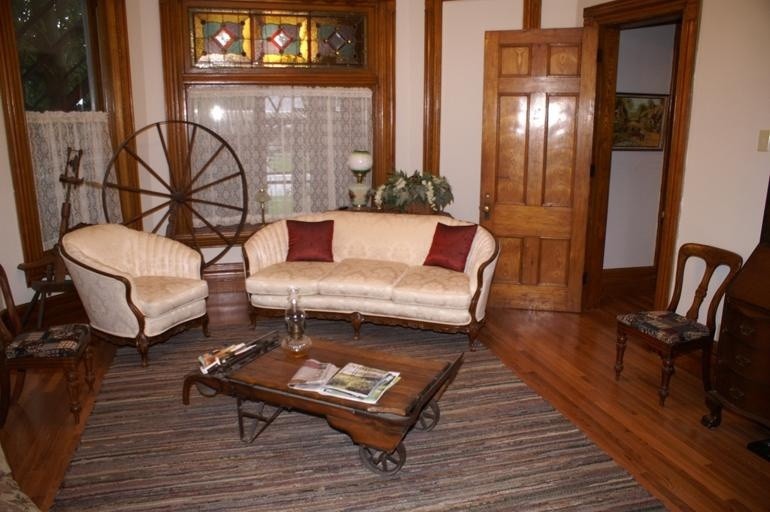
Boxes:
[49,319,666,512]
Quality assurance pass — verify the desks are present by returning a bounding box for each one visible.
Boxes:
[335,208,450,216]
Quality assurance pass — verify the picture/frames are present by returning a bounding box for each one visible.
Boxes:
[611,93,668,151]
[384,202,432,214]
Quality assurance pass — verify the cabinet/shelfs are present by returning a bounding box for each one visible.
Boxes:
[703,179,770,458]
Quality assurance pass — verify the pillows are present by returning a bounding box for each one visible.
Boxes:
[423,222,477,273]
[286,219,334,261]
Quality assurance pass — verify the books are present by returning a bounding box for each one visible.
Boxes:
[287,359,403,405]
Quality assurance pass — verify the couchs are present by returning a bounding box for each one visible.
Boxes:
[242,210,500,352]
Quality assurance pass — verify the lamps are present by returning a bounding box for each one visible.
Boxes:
[255,188,271,228]
[346,150,373,210]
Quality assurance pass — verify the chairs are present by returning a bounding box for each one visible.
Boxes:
[0,264,95,429]
[615,242,742,405]
[57,223,209,368]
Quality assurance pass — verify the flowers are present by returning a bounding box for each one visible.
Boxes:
[373,168,454,210]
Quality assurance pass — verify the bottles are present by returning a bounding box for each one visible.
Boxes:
[280,287,313,360]
